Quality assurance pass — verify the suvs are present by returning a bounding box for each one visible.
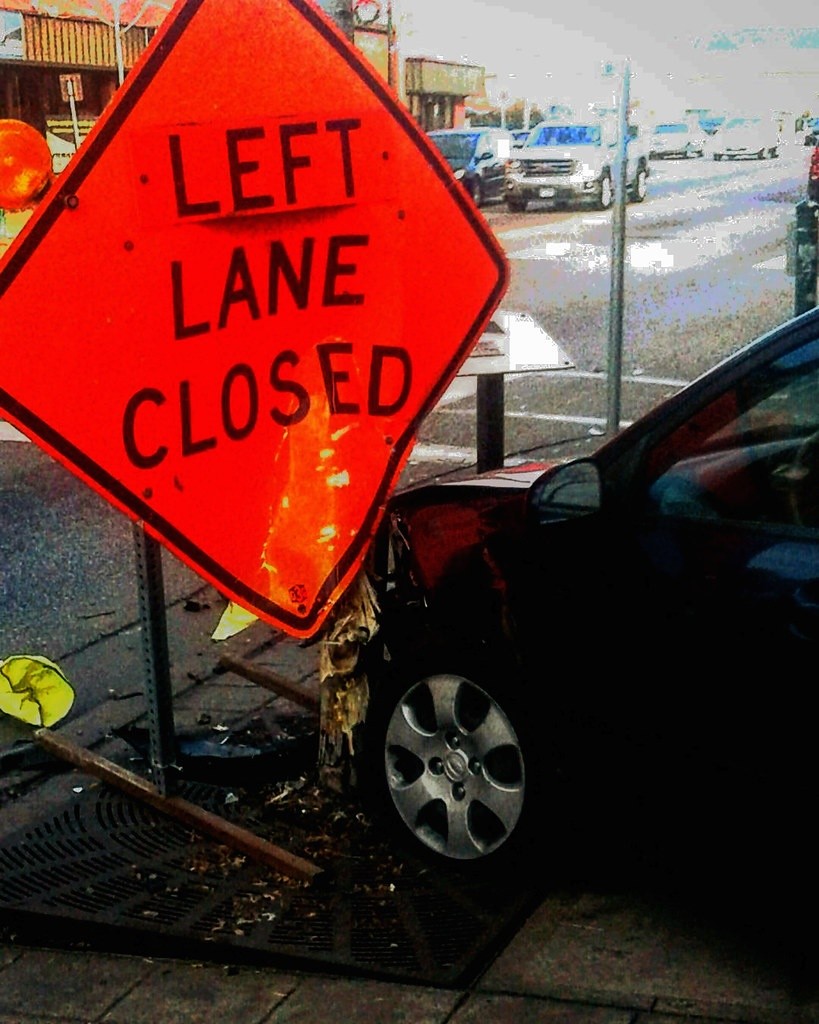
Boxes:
[502,119,652,212]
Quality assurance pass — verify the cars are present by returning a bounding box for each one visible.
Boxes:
[357,302,819,1004]
[789,137,819,315]
[803,117,819,147]
[706,119,780,161]
[651,123,705,159]
[422,125,514,208]
